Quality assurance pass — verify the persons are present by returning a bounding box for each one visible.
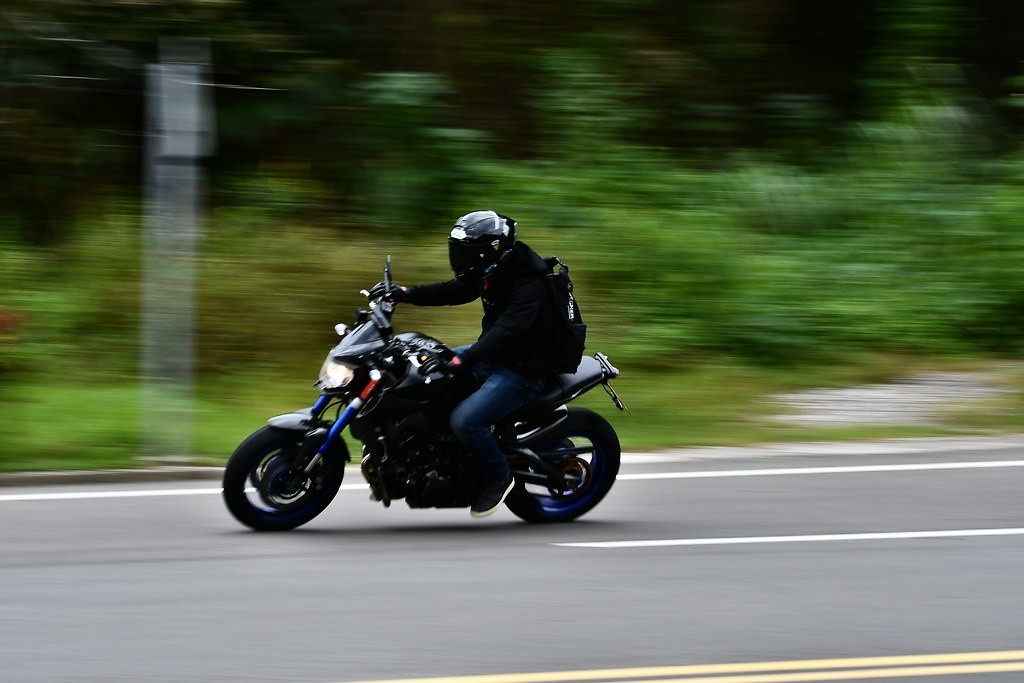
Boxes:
[366,209,569,516]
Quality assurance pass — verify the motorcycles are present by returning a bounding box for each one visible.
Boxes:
[222,255,633,532]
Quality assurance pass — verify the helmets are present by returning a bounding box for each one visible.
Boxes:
[450,210,517,277]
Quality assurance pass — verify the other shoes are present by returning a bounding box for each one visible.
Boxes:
[470,476,515,518]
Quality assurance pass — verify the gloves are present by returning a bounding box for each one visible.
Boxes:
[417,350,457,376]
[369,283,406,304]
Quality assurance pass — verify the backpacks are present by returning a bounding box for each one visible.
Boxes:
[535,253,587,375]
